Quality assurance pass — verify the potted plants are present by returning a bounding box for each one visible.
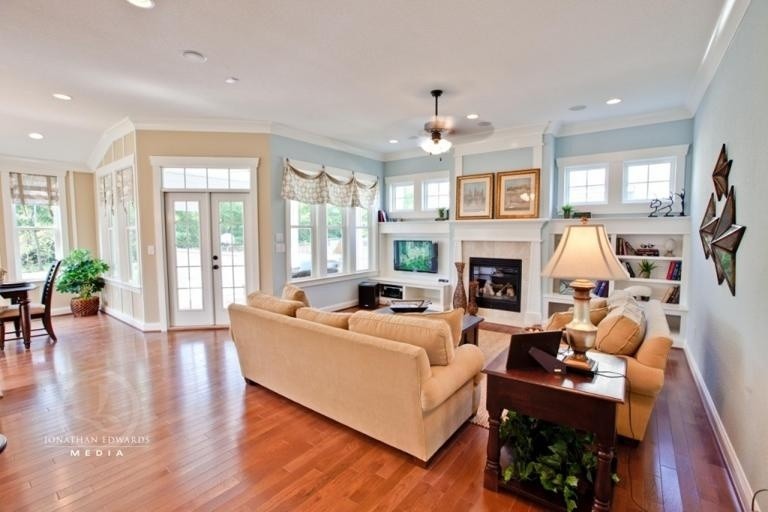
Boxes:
[561,204,574,218]
[56,248,110,320]
[637,260,657,278]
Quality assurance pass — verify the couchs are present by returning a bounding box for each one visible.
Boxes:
[227,284,489,471]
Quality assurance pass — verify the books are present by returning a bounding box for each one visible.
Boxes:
[593,280,607,297]
[617,238,637,255]
[667,261,680,280]
[661,284,679,303]
[620,258,635,277]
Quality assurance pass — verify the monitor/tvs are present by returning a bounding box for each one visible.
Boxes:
[394,240,438,273]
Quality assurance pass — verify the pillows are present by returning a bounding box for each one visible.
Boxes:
[591,295,608,310]
[407,308,465,348]
[279,285,310,305]
[547,307,609,329]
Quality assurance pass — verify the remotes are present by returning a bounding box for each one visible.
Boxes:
[438,279,448,282]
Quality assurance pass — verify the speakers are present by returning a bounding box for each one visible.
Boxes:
[359,281,379,309]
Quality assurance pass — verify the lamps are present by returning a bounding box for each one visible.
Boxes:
[419,136,453,158]
[539,214,635,377]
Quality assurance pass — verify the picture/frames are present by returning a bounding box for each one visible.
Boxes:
[505,330,567,378]
[454,172,495,221]
[493,168,541,220]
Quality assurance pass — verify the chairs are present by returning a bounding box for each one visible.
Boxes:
[536,297,674,449]
[1,258,62,352]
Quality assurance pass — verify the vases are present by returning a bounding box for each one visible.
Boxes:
[466,280,480,315]
[452,261,468,315]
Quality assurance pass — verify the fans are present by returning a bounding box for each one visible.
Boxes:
[380,90,495,148]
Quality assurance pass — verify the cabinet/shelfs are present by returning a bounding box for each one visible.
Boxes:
[369,277,451,313]
[545,216,690,349]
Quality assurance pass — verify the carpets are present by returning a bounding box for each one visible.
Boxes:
[470,326,517,431]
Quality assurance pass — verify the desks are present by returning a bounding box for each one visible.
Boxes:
[0,281,38,350]
[479,336,629,512]
